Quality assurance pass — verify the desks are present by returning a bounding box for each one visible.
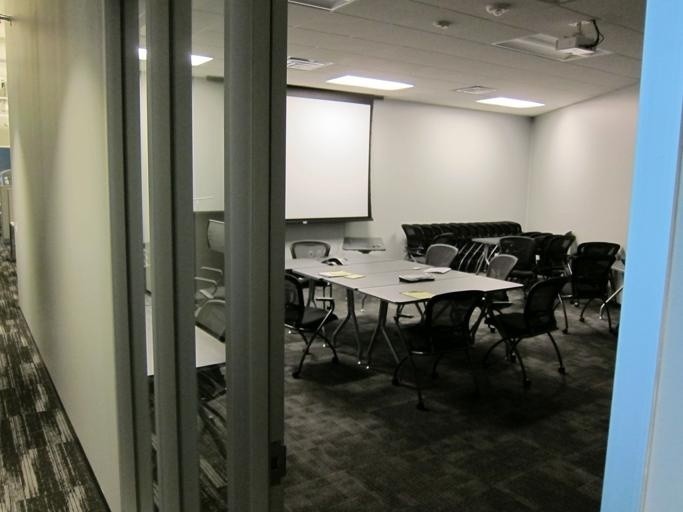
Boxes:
[285,258,434,347]
[358,273,523,370]
[145,289,226,435]
[284,257,332,335]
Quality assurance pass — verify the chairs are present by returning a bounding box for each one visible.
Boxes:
[400,220,625,335]
[392,289,490,411]
[393,244,458,326]
[464,254,519,344]
[482,273,574,387]
[283,273,340,378]
[286,242,334,334]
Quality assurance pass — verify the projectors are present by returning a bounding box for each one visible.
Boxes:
[555,35,596,55]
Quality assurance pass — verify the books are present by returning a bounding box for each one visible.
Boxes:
[425,266,452,275]
[399,272,435,283]
[318,270,350,279]
